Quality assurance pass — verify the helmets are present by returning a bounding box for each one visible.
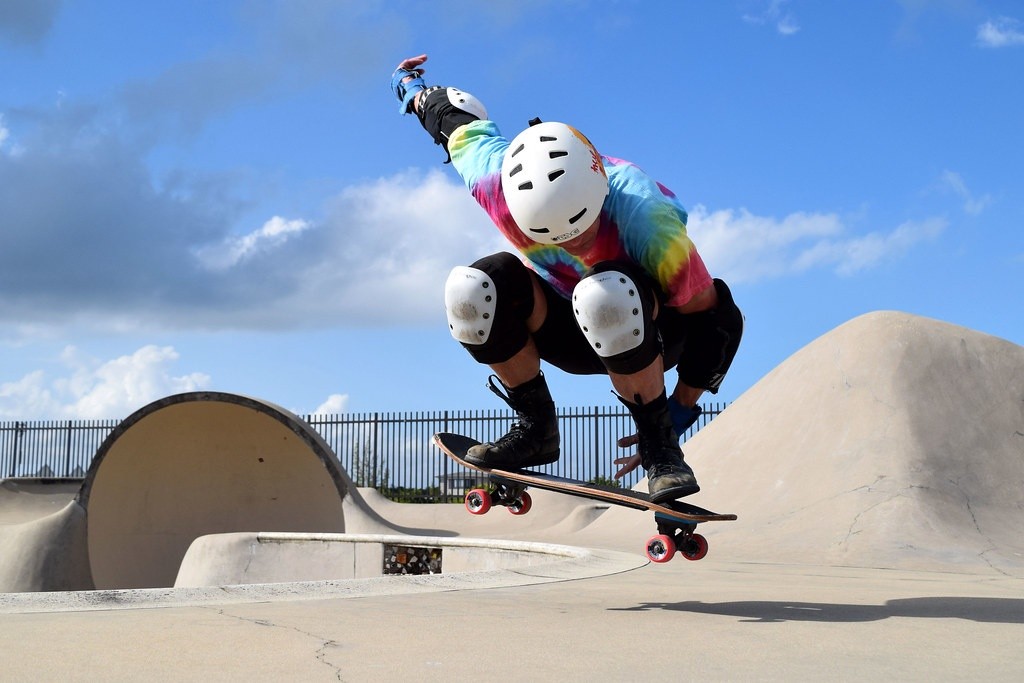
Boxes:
[501,122,609,244]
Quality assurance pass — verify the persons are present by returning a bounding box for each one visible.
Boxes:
[390,54,743,503]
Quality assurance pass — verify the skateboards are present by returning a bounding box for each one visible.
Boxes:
[430,428,741,566]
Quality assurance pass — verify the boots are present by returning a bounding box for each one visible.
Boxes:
[464,370,560,467]
[611,386,700,503]
